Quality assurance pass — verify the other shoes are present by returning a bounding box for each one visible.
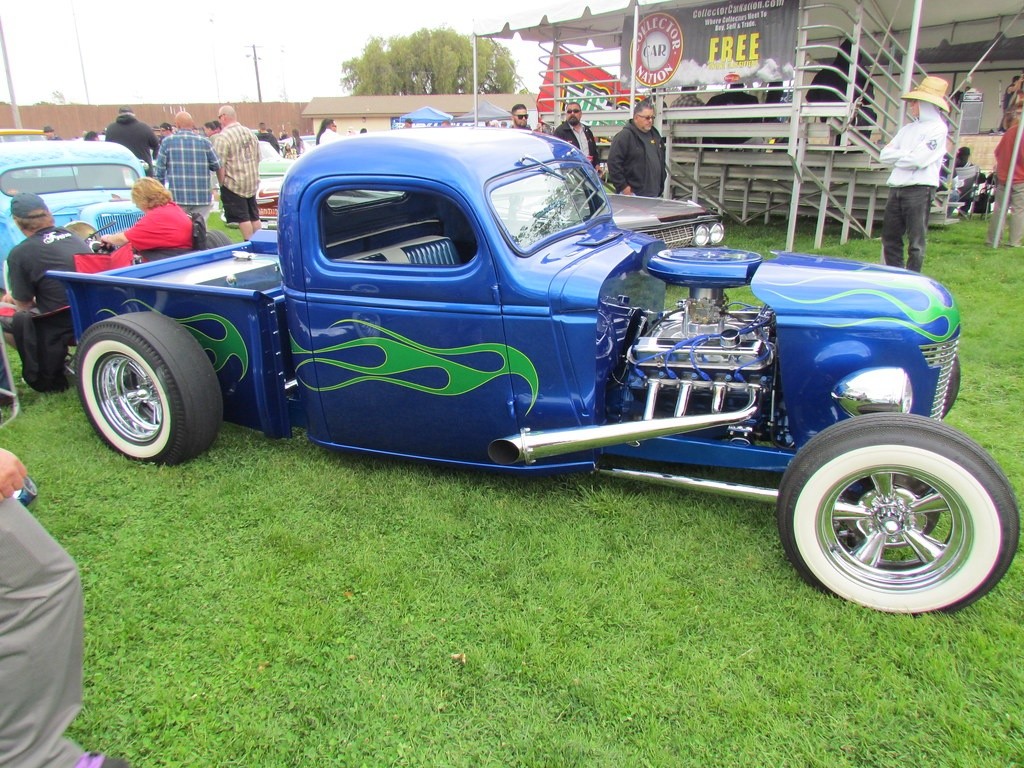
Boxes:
[74,752,128,768]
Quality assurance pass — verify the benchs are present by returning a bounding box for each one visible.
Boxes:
[329,234,462,268]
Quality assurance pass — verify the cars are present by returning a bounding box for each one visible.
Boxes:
[256,140,297,199]
[0,141,147,295]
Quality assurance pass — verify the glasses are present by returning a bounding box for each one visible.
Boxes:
[407,122,411,124]
[567,109,581,114]
[514,114,528,119]
[218,114,225,119]
[637,115,655,120]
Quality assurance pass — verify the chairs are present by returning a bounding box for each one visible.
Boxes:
[13,242,135,376]
[951,164,1001,221]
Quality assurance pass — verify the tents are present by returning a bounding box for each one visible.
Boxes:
[452,101,513,128]
[400,106,453,127]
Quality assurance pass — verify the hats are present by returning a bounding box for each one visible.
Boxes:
[44,126,54,132]
[9,192,48,218]
[501,122,507,125]
[900,77,950,113]
[405,119,413,123]
[160,123,173,130]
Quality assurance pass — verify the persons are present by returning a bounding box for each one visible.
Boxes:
[705,83,763,152]
[608,101,668,197]
[806,37,877,152]
[214,106,261,242]
[670,86,706,151]
[646,88,668,108]
[0,449,131,768]
[934,77,1024,246]
[553,102,605,177]
[511,104,533,131]
[44,118,507,158]
[157,112,224,224]
[105,106,160,177]
[879,76,950,274]
[100,177,193,264]
[0,193,94,350]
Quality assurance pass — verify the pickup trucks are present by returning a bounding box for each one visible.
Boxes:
[42,124,1020,612]
